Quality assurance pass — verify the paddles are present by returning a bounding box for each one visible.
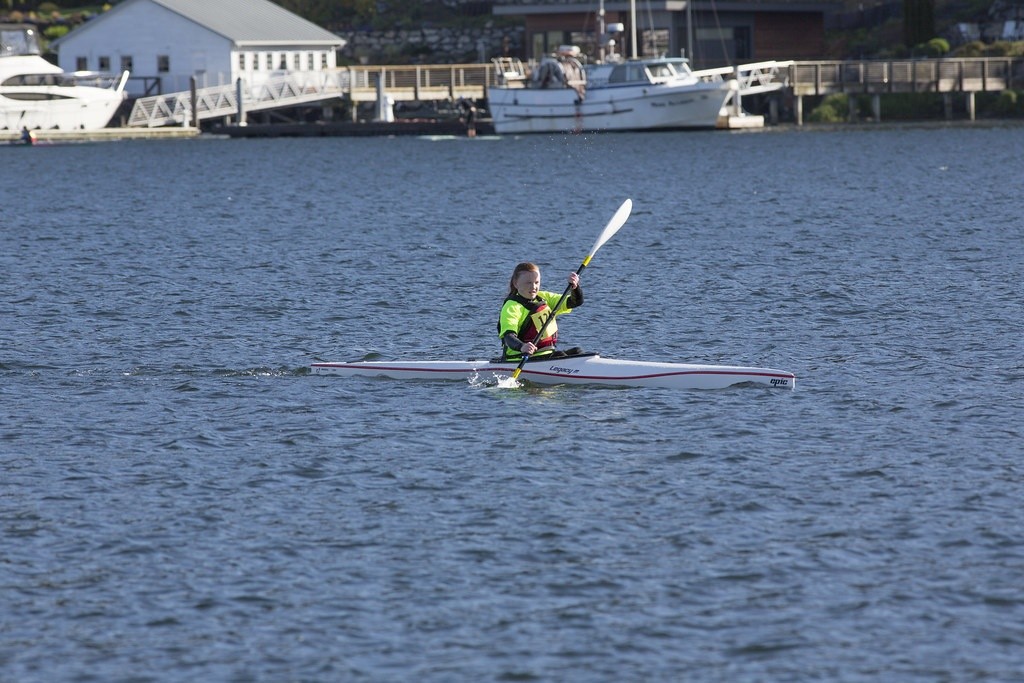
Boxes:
[511,197,634,380]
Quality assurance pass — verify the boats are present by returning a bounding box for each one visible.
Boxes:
[483,0,745,134]
[0,53,130,140]
[310,353,798,395]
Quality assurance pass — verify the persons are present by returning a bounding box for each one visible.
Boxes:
[459,97,477,137]
[497,262,584,360]
